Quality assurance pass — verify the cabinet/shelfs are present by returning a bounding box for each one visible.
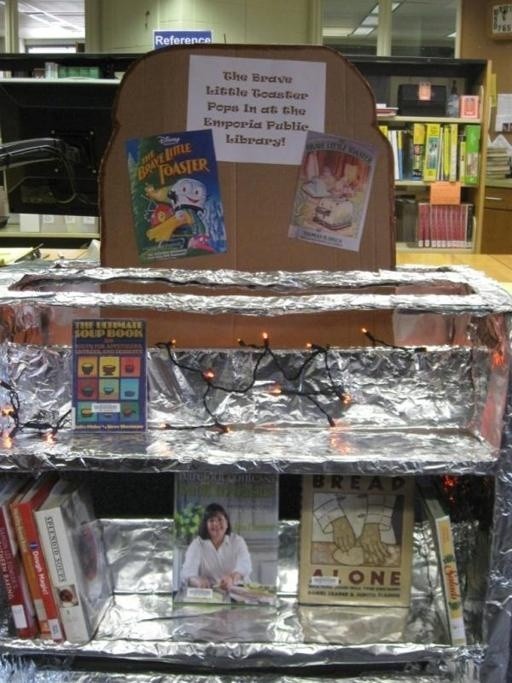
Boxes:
[480,185,512,255]
[0,53,146,83]
[0,266,510,680]
[344,54,493,255]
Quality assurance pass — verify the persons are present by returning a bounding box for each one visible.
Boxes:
[311,487,400,566]
[182,501,254,591]
[415,475,466,648]
[1,476,40,636]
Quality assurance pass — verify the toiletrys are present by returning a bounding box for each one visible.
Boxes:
[446,80,459,117]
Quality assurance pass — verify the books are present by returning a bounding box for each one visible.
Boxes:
[72,318,147,433]
[486,165,510,170]
[298,462,414,607]
[486,148,508,150]
[449,124,458,181]
[486,170,511,175]
[437,128,443,180]
[173,469,279,604]
[398,131,403,181]
[32,475,112,644]
[459,135,467,183]
[486,155,509,158]
[441,140,443,180]
[486,150,506,155]
[464,125,481,183]
[486,158,509,161]
[486,161,507,165]
[376,103,399,117]
[9,477,51,632]
[18,476,65,642]
[444,125,449,181]
[391,130,399,179]
[407,123,426,180]
[423,123,441,181]
[415,198,475,252]
[486,175,505,179]
